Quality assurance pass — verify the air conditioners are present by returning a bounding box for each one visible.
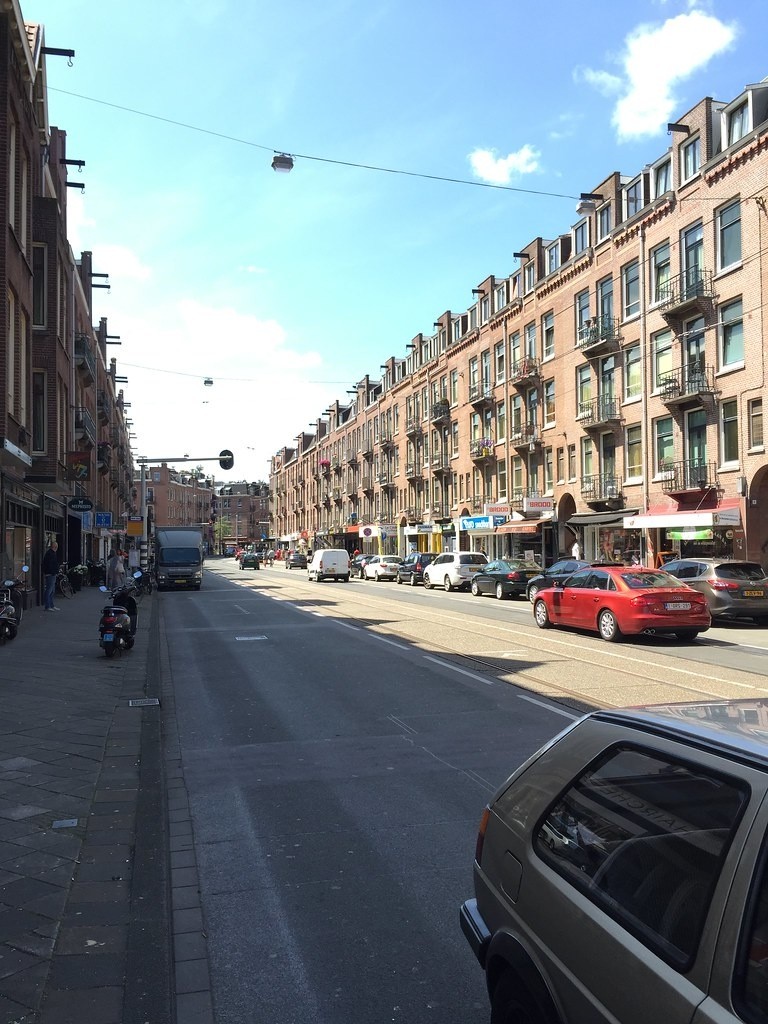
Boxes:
[607,486,618,499]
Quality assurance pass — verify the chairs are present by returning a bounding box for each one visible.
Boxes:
[665,379,680,400]
[687,369,709,393]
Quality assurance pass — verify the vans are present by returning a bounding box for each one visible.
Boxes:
[307,549,353,582]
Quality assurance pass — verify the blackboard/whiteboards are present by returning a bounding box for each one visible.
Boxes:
[654,551,680,569]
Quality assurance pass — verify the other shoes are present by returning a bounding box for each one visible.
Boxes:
[45,607,61,612]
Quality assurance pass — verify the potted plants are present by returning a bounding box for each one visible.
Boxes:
[439,396,448,405]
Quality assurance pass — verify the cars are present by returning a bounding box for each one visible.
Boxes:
[350,553,377,578]
[471,559,543,600]
[525,559,627,604]
[236,551,263,570]
[363,555,402,582]
[533,565,711,643]
[285,554,307,569]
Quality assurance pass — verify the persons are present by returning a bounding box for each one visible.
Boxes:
[572,539,581,560]
[107,548,129,591]
[43,541,60,611]
[479,546,487,558]
[263,548,312,567]
[354,547,361,555]
[210,543,218,555]
[604,527,625,561]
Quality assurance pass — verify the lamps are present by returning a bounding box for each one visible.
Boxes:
[459,374,464,378]
[18,429,28,446]
[557,432,567,438]
[184,453,189,458]
[204,377,213,387]
[272,151,296,174]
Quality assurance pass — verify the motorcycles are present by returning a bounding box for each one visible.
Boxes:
[0,565,30,644]
[126,570,156,598]
[98,571,143,658]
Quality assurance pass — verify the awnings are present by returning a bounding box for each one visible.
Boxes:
[495,519,552,533]
[623,507,741,528]
[565,512,637,526]
[264,534,300,541]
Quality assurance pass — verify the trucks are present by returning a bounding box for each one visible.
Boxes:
[154,525,205,591]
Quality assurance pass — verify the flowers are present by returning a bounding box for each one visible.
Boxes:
[319,459,330,467]
[73,564,89,574]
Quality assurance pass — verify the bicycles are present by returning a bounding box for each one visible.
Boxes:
[57,558,105,600]
[270,557,274,567]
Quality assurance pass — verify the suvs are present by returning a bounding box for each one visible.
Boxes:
[396,551,441,586]
[459,698,768,1024]
[224,547,235,557]
[658,558,768,625]
[423,551,490,592]
